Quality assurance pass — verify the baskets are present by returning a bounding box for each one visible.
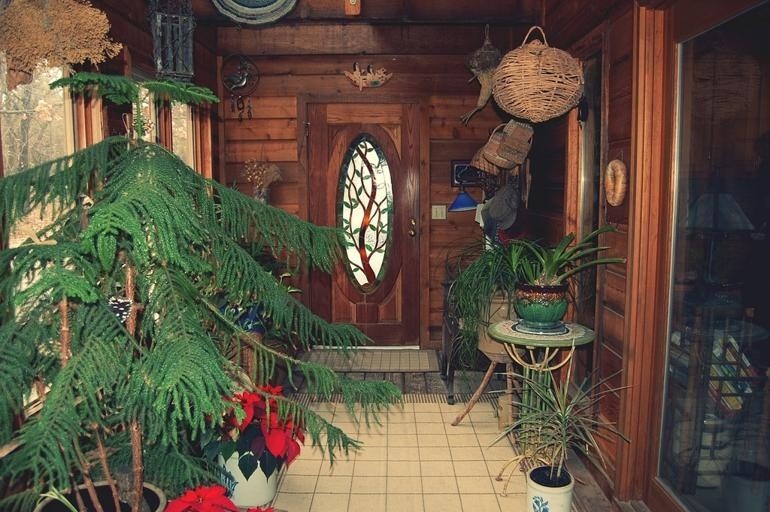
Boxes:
[492,41,583,125]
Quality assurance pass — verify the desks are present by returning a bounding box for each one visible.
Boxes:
[489,320,597,482]
[660,282,759,496]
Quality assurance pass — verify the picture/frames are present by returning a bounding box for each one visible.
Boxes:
[450,159,484,187]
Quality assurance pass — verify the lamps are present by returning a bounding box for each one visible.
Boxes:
[447,176,488,212]
[682,191,755,287]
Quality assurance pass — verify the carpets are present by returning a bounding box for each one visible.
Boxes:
[295,349,439,373]
[229,393,587,512]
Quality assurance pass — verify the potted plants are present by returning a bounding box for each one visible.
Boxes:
[439,232,528,432]
[503,223,628,338]
[0,69,405,512]
[511,360,629,512]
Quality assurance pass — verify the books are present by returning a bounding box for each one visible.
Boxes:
[668,325,760,416]
